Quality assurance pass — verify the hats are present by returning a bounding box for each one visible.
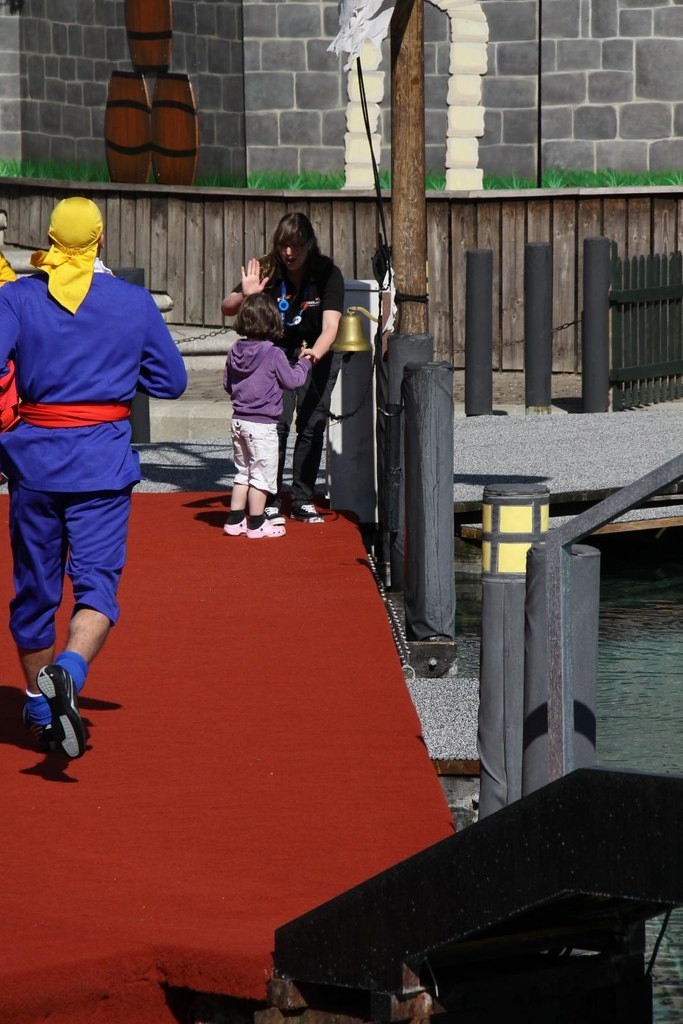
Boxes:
[30,196,104,316]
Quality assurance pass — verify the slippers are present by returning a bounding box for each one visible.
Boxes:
[246,522,286,538]
[223,517,247,536]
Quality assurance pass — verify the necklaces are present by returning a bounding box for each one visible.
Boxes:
[280,279,310,327]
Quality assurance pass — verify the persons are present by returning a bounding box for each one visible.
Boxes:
[220,211,345,526]
[222,291,313,539]
[0,196,188,759]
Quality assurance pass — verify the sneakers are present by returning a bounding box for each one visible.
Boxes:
[290,505,325,523]
[21,664,87,760]
[263,507,286,525]
